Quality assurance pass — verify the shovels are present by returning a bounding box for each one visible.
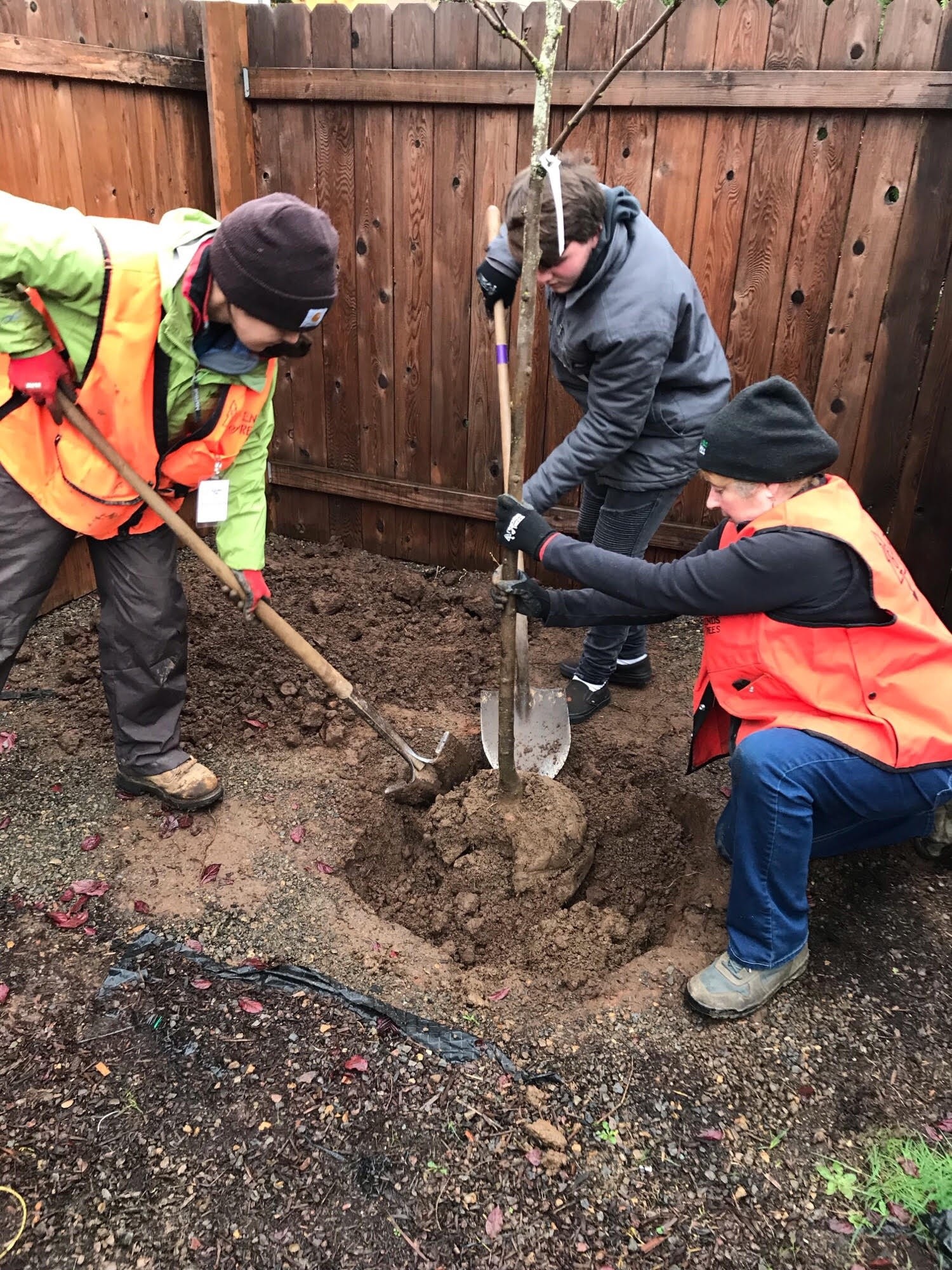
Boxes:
[55,384,473,808]
[480,205,572,780]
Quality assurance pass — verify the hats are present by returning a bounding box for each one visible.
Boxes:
[697,376,839,483]
[210,192,340,331]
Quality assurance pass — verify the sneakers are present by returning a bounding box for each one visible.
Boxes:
[559,658,652,684]
[686,942,809,1019]
[917,799,952,859]
[118,757,224,808]
[567,680,611,721]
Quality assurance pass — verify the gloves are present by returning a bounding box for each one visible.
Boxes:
[9,347,77,426]
[495,495,553,561]
[491,563,550,622]
[476,259,516,318]
[221,571,270,628]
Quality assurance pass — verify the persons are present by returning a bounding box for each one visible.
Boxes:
[475,150,735,725]
[0,190,340,814]
[490,373,952,1025]
